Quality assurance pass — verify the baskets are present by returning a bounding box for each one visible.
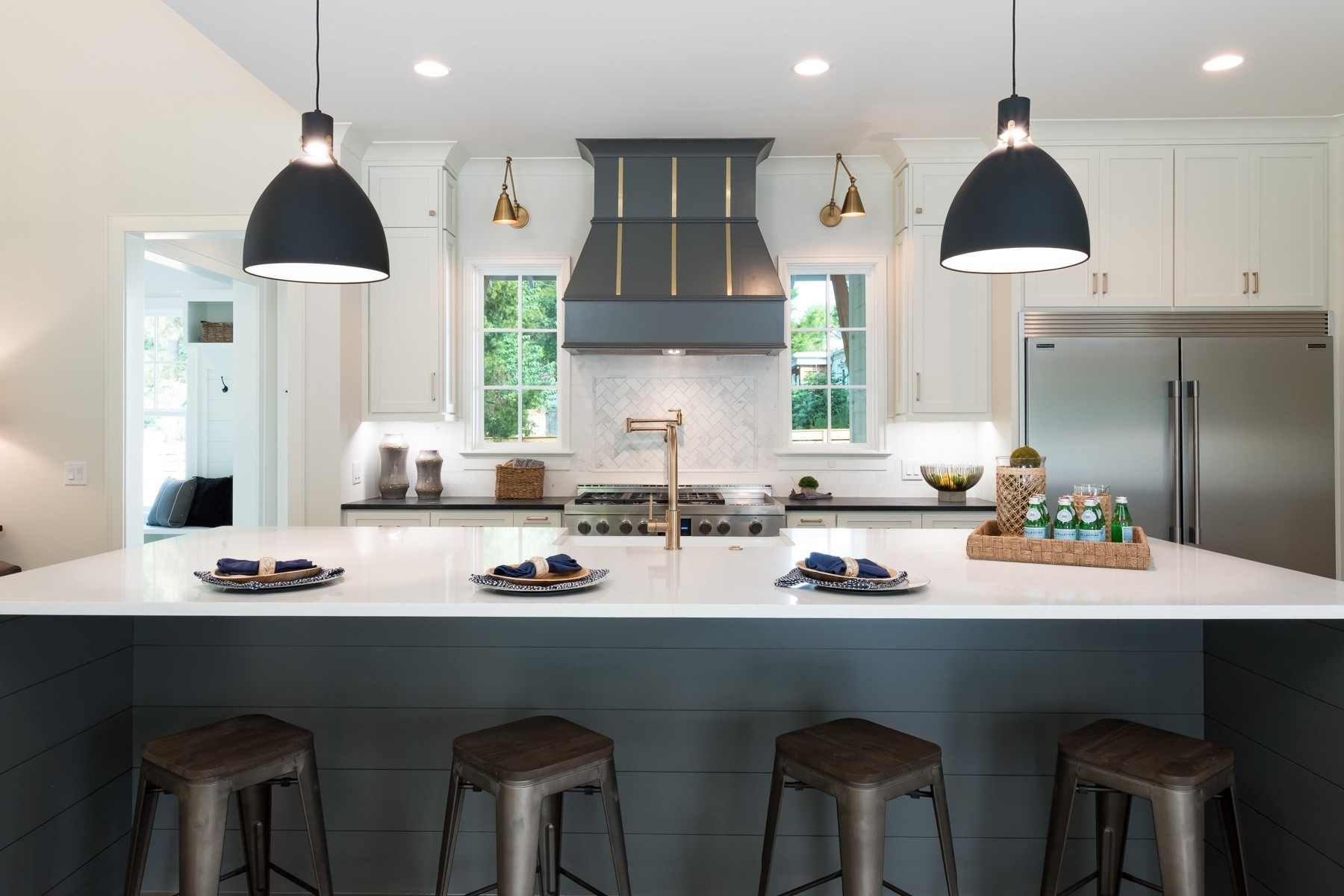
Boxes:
[966,519,1150,569]
[202,322,233,342]
[496,459,545,500]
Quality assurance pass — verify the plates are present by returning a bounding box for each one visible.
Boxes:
[202,567,342,589]
[796,561,931,592]
[472,564,607,592]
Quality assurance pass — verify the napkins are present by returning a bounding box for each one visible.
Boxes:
[217,558,317,576]
[493,553,582,578]
[805,552,892,579]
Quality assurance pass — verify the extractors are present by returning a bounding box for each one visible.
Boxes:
[561,137,786,356]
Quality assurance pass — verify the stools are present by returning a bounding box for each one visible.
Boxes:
[1040,717,1251,896]
[127,712,334,896]
[759,717,959,896]
[434,715,630,896]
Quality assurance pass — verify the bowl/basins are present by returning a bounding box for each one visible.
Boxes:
[920,463,984,501]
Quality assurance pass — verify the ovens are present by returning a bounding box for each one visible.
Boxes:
[565,515,787,537]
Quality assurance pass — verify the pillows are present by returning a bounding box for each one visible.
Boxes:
[146,475,233,528]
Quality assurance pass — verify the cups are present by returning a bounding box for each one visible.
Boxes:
[995,455,1047,537]
[1074,482,1112,541]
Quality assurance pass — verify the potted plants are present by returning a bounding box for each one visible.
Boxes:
[798,476,818,495]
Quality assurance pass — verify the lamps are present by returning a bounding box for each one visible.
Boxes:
[243,0,390,285]
[491,156,529,228]
[940,0,1090,273]
[820,152,866,227]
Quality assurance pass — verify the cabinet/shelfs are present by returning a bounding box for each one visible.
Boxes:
[183,297,234,479]
[783,510,1000,530]
[882,133,1329,421]
[344,510,566,527]
[352,163,460,425]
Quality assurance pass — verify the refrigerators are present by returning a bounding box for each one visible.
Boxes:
[1017,311,1336,583]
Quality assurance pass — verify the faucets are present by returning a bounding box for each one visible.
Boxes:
[645,425,682,550]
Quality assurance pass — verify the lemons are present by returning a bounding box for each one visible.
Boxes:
[930,473,977,489]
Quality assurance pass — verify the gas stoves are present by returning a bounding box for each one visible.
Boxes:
[564,483,787,516]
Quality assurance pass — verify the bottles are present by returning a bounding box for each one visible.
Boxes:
[1024,492,1107,542]
[1111,496,1133,544]
[378,432,410,500]
[415,450,444,500]
